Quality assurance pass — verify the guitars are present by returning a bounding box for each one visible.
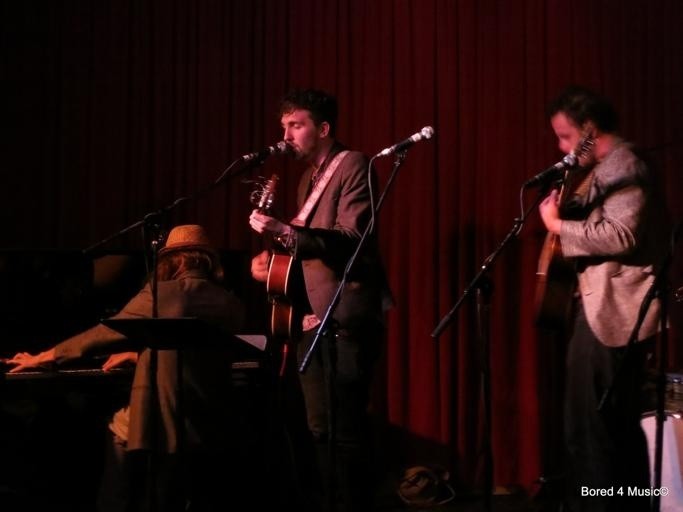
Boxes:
[529,126,595,328]
[251,175,306,379]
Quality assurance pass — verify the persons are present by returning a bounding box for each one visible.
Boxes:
[533,81,678,512]
[245,81,383,510]
[1,219,250,511]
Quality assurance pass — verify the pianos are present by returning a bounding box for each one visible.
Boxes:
[0,354,262,413]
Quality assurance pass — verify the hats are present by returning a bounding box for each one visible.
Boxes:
[159,225,217,256]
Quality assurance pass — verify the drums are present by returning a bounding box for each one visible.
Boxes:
[644,371,681,413]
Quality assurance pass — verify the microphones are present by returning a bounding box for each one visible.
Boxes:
[522,154,571,190]
[239,141,288,164]
[376,126,435,159]
